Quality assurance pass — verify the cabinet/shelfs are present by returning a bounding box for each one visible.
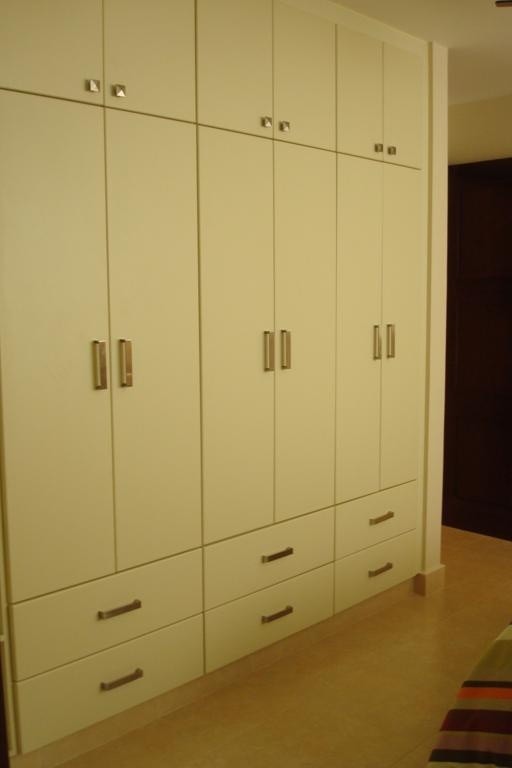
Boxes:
[337,470,426,622]
[337,151,427,506]
[204,502,336,682]
[2,0,197,124]
[336,0,431,172]
[0,547,204,759]
[199,122,337,551]
[0,88,200,611]
[195,0,336,150]
